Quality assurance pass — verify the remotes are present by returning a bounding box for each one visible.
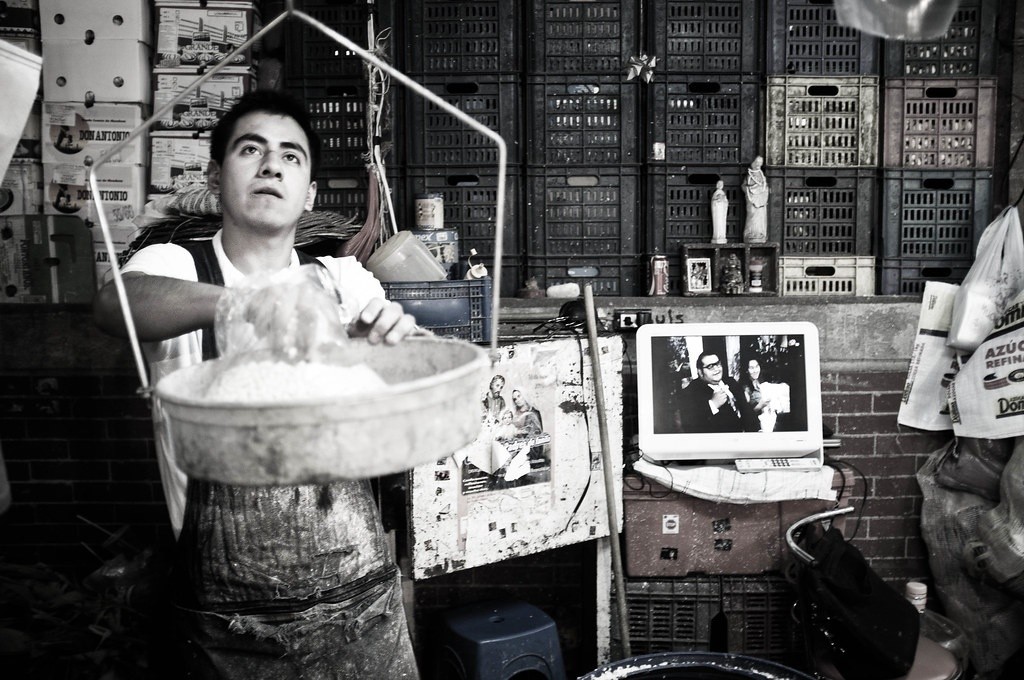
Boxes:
[733,457,821,475]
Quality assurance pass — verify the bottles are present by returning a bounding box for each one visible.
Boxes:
[463,263,488,279]
[900,579,971,680]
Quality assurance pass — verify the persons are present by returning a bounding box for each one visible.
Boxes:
[483,375,542,441]
[681,350,778,432]
[742,154,769,233]
[92,88,434,680]
[712,181,728,238]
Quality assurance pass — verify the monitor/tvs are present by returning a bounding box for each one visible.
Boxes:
[637,322,823,466]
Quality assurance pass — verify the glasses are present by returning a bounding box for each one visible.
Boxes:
[703,360,722,370]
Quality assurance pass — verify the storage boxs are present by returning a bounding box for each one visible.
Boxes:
[612,467,855,657]
[0,1,998,346]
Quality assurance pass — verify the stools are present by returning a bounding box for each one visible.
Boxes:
[440,604,567,680]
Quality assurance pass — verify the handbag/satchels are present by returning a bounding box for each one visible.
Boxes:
[934,435,1008,498]
[945,201,1024,353]
